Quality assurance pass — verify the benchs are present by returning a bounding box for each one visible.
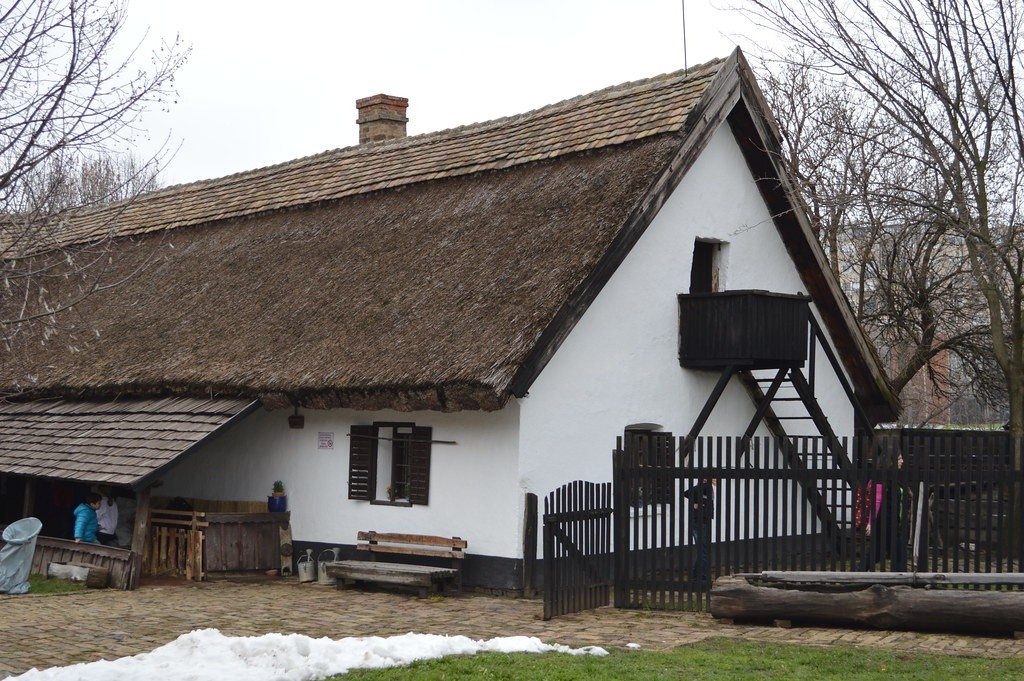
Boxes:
[326,530,468,598]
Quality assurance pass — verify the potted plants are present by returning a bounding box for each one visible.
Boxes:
[271,480,284,496]
[387,486,396,502]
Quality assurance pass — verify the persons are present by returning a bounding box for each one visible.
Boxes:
[94,484,119,546]
[74,493,106,545]
[0,504,48,536]
[111,484,136,548]
[856,446,904,572]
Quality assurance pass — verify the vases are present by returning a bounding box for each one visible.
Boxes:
[267,495,286,512]
